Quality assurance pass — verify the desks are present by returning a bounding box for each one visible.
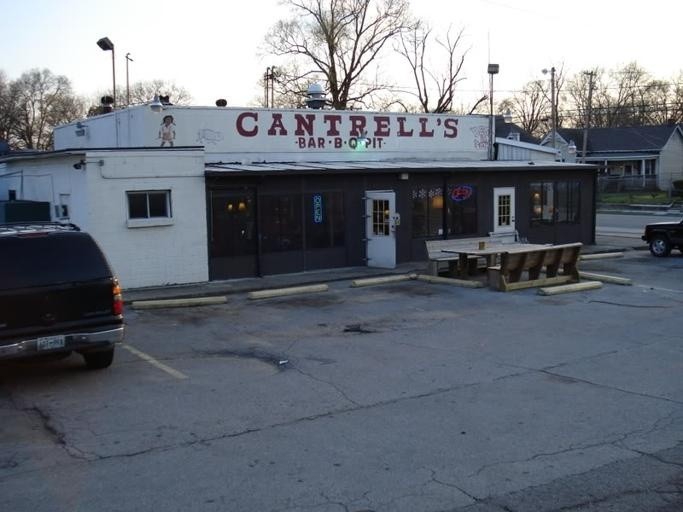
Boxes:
[441,244,552,278]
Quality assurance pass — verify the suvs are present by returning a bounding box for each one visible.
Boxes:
[0,219,125,368]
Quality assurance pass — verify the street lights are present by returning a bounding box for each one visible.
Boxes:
[487,63,499,160]
[542,67,556,148]
[125,52,133,105]
[265,65,278,108]
[96,37,117,109]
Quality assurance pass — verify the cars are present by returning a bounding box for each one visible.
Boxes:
[641,216,683,257]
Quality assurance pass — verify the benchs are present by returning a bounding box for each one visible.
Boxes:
[488,230,530,244]
[425,237,503,276]
[488,242,583,292]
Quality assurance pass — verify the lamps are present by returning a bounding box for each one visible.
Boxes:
[150,103,167,113]
[73,160,104,170]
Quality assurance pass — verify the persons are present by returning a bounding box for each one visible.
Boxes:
[155,112,177,148]
